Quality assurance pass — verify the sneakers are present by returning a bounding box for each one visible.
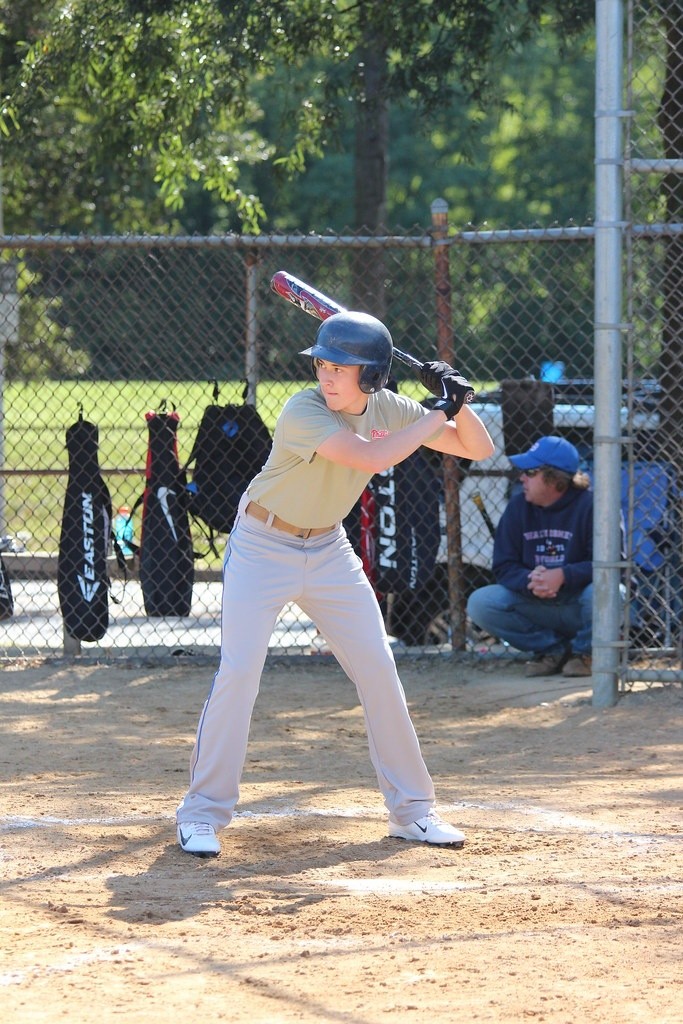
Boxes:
[388,809,466,848]
[176,822,221,857]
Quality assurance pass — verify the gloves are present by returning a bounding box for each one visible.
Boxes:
[432,370,475,422]
[411,360,454,398]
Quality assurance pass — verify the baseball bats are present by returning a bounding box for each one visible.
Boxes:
[470,490,547,663]
[267,268,477,406]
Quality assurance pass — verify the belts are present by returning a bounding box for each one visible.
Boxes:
[245,501,336,539]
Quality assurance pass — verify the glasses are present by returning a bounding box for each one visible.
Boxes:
[519,466,548,477]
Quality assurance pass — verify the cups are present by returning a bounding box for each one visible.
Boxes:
[540,361,565,382]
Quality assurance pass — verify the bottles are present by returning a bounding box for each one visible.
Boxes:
[115,507,133,557]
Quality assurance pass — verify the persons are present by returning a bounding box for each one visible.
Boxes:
[174,311,495,855]
[466,436,628,678]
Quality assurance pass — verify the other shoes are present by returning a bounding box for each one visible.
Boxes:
[562,652,591,676]
[525,652,562,677]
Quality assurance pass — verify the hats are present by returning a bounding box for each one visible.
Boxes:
[507,436,580,476]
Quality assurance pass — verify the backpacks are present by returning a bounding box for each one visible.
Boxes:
[176,403,272,536]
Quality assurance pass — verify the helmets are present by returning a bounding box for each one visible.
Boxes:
[299,311,393,394]
